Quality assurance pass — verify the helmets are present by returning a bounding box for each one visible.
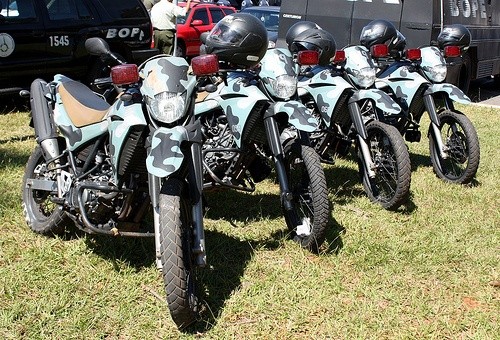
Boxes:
[286,22,323,52]
[437,24,471,51]
[207,13,268,71]
[359,19,397,50]
[291,29,336,66]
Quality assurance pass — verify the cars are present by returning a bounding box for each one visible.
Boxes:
[0,1,19,17]
[151,2,237,61]
[238,5,282,42]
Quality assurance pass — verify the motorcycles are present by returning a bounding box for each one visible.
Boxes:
[20,36,261,334]
[194,44,480,249]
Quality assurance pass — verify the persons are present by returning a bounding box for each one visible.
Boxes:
[151,0,191,54]
[259,0,269,7]
[241,0,253,10]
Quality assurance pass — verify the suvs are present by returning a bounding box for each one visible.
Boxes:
[0,0,159,92]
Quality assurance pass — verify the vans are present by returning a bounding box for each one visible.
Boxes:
[276,0,500,99]
